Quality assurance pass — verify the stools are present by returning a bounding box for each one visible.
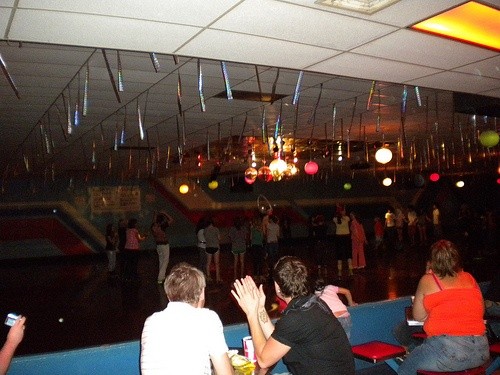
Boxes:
[352,332,500,375]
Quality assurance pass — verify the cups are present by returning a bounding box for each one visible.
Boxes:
[243,336,257,363]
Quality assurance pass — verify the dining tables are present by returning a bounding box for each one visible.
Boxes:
[228,347,278,375]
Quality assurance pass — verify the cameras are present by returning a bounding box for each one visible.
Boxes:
[5,314,21,327]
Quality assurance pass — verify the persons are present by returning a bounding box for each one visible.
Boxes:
[105,202,441,285]
[141,263,233,375]
[312,274,359,339]
[230,256,355,375]
[397,239,489,375]
[0,315,26,375]
